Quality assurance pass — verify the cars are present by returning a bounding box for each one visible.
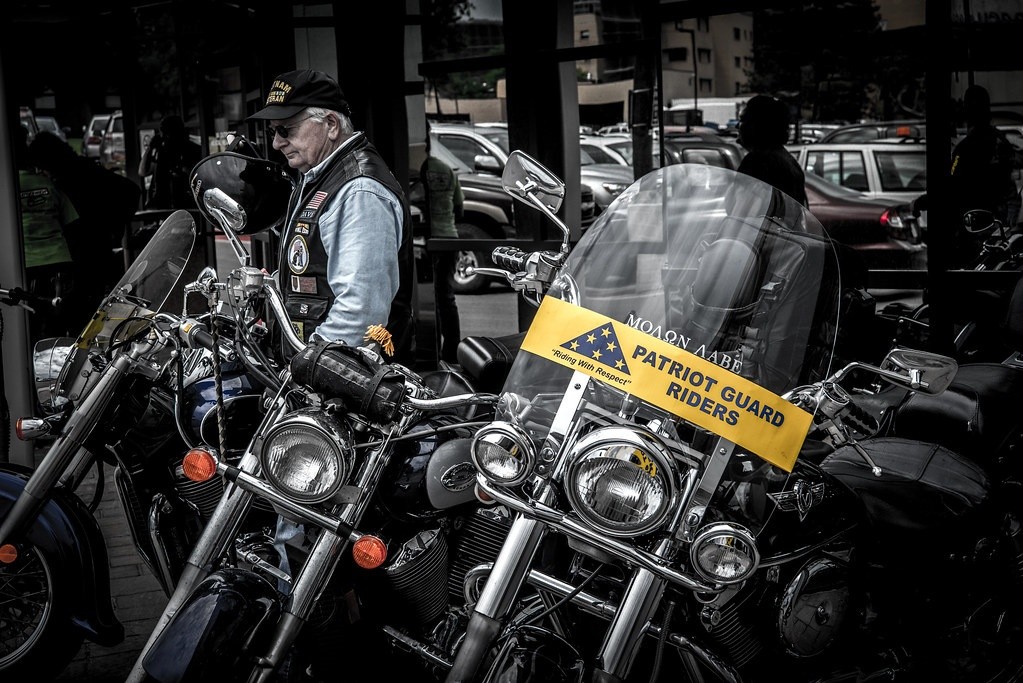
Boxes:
[21,103,154,174]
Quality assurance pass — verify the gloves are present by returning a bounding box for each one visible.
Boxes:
[258,364,306,416]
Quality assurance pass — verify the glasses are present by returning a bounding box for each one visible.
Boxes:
[268,112,325,138]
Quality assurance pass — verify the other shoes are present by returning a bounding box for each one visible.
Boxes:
[441,356,460,367]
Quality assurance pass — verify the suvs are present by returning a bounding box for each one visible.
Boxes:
[430,121,1022,293]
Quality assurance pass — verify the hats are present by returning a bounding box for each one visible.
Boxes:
[245,68,349,123]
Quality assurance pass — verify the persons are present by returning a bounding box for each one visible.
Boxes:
[243,70,413,361]
[424,117,466,364]
[840,77,880,123]
[137,116,201,209]
[950,85,1016,258]
[11,131,140,321]
[736,95,804,206]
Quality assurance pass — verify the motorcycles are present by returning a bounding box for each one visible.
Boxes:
[0,187,1021,683]
